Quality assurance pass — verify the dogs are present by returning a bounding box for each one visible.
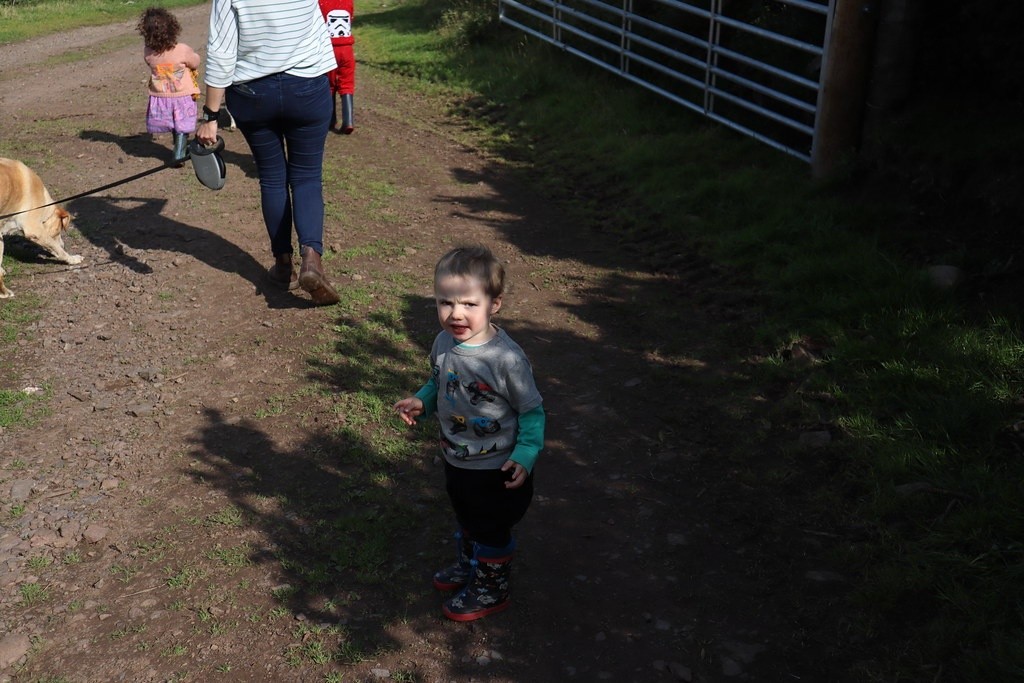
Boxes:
[0,158,84,298]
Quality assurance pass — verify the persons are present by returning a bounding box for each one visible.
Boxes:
[134,5,201,166]
[392,242,546,621]
[318,0,355,134]
[195,0,341,307]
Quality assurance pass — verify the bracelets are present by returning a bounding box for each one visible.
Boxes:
[202,104,220,116]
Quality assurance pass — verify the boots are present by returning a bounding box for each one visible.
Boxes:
[299,245,339,305]
[170,133,187,168]
[434,526,477,591]
[267,253,299,289]
[340,94,353,134]
[328,94,336,129]
[442,537,513,621]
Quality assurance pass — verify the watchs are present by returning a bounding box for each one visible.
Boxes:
[203,112,219,122]
